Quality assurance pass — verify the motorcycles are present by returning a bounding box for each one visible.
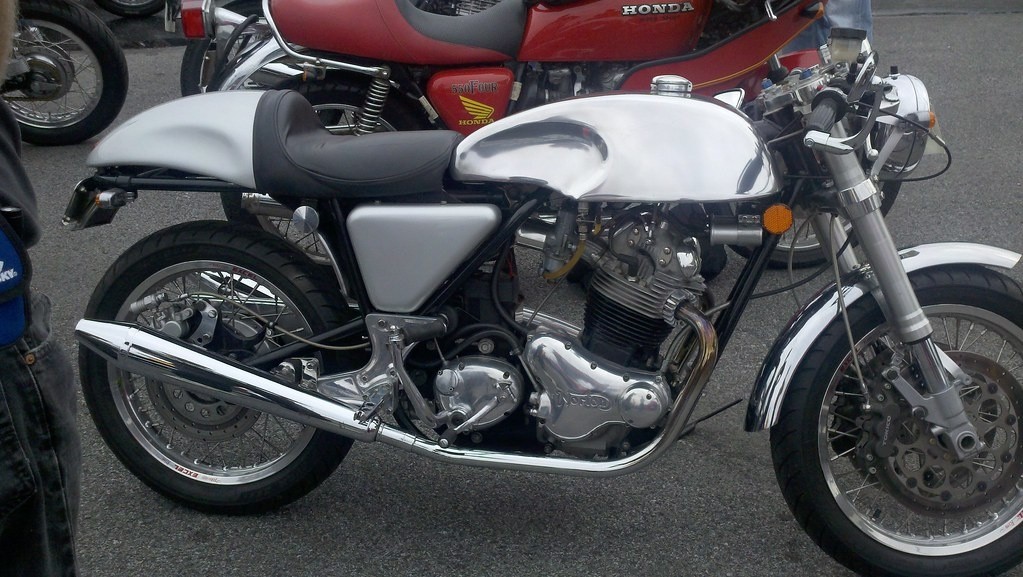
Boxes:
[0,0,905,577]
[51,1,1023,577]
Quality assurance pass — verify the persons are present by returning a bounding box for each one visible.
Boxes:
[0,93,82,577]
